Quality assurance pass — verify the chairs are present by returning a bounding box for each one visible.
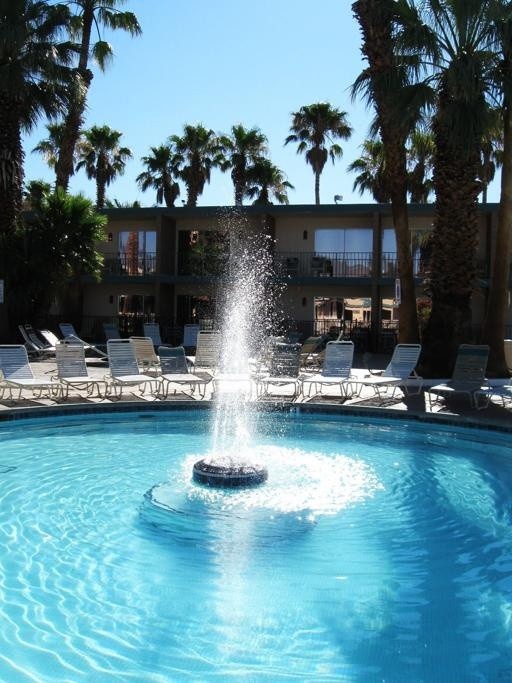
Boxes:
[261,326,512,412]
[0,319,223,404]
